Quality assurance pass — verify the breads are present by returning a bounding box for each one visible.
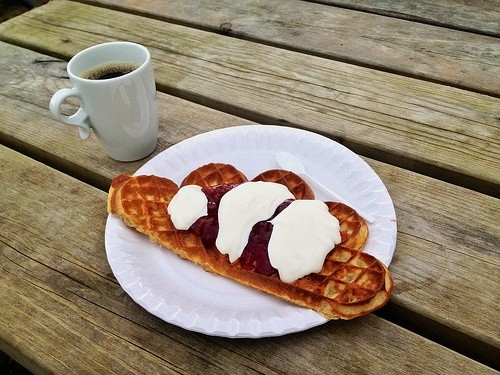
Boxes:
[107,164,394,321]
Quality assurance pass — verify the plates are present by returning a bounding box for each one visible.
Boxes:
[104,125,398,338]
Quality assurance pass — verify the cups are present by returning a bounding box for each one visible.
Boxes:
[49,42,158,161]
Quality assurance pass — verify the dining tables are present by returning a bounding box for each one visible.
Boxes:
[0,0,500,374]
